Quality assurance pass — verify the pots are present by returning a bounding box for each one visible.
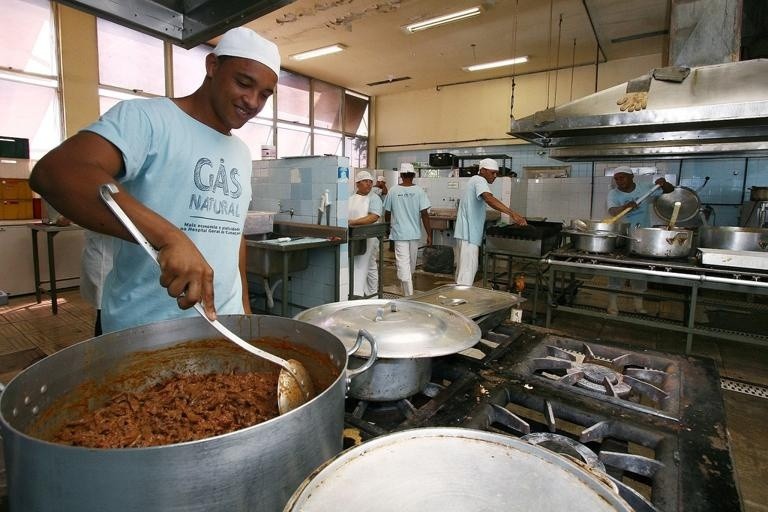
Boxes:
[346,356,435,402]
[568,220,766,263]
[748,184,768,200]
[284,420,640,512]
[2,314,375,512]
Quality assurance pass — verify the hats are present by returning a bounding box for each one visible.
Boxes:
[613,166,633,176]
[354,170,374,183]
[378,175,387,183]
[399,162,417,174]
[477,158,500,175]
[209,25,282,79]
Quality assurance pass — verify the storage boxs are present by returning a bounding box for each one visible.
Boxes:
[243,209,278,236]
[0,135,46,221]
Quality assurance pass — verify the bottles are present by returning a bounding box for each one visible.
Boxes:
[261,143,277,161]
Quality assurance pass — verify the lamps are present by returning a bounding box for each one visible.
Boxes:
[457,40,534,74]
[397,3,485,34]
[286,41,349,63]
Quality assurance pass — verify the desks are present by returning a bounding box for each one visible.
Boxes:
[20,220,88,316]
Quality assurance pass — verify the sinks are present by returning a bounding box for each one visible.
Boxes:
[243,232,299,242]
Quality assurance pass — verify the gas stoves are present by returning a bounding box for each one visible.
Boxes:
[358,318,661,512]
[543,242,767,285]
[483,231,562,258]
[340,316,741,512]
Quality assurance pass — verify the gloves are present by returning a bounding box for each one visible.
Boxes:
[617,77,651,112]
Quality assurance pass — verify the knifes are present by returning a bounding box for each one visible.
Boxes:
[278,236,303,242]
[317,195,325,225]
[323,189,331,226]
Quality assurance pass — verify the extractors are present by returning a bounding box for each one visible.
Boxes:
[512,0,767,160]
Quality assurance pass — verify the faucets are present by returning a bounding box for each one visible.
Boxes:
[280,208,295,218]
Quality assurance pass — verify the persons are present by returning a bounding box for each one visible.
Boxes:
[373,175,388,196]
[605,164,674,316]
[382,161,433,298]
[452,157,527,287]
[26,23,281,337]
[346,169,383,298]
[53,212,115,337]
